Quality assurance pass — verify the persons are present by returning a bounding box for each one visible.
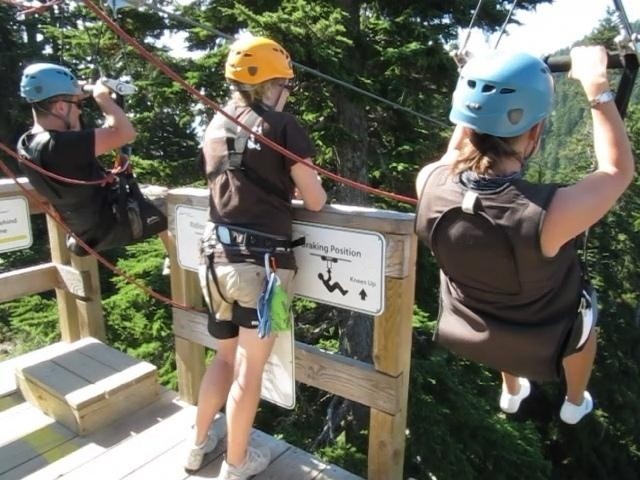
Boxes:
[181,33,328,479]
[411,44,637,426]
[14,62,173,256]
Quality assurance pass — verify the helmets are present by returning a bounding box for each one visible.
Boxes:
[18,60,83,105]
[224,37,294,89]
[448,44,556,139]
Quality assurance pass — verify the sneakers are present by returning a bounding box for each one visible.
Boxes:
[182,427,220,473]
[556,385,593,424]
[212,444,272,480]
[498,373,531,415]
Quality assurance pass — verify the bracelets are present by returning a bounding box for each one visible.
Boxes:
[588,89,615,110]
